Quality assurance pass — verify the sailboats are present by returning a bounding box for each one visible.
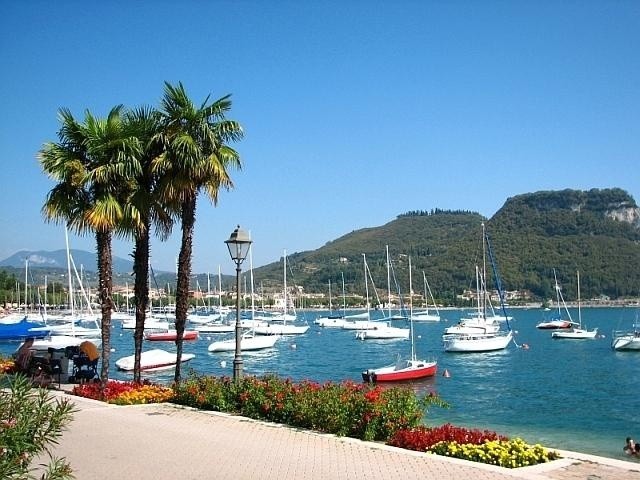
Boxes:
[443,220,513,352]
[314,244,441,338]
[1,222,134,352]
[120,249,309,352]
[551,270,598,338]
[363,256,438,382]
[536,267,578,329]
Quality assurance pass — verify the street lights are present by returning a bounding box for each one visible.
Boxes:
[224,225,253,380]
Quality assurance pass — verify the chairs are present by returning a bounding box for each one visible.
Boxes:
[12,348,101,388]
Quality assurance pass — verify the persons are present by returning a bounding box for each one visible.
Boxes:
[19,336,34,355]
[623,436,640,456]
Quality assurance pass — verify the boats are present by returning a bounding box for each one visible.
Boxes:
[611,322,640,350]
[115,348,195,371]
